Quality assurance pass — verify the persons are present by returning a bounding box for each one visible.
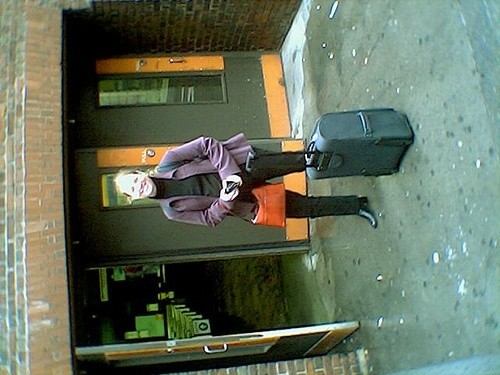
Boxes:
[115,133,379,230]
